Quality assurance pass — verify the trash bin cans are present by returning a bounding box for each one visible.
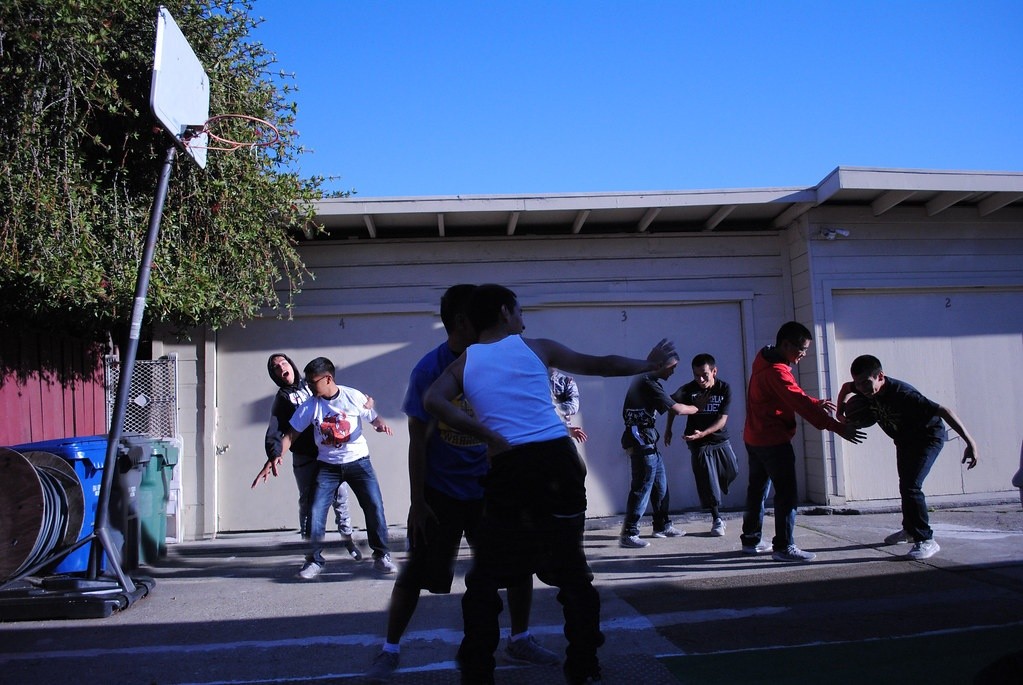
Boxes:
[107,431,151,570]
[140,437,179,562]
[7,435,107,574]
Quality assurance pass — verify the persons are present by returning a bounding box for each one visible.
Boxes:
[740,321,867,562]
[366,284,558,685]
[664,353,739,536]
[264,353,374,561]
[837,355,976,558]
[549,369,588,443]
[252,357,399,580]
[618,351,711,549]
[423,284,677,685]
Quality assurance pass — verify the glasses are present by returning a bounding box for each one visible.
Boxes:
[303,376,330,386]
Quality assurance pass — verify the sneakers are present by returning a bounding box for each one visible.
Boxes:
[711,518,726,536]
[373,555,398,573]
[294,561,321,581]
[884,528,924,545]
[502,635,561,668]
[362,647,400,684]
[652,527,687,538]
[772,544,817,563]
[618,535,648,548]
[742,540,775,553]
[905,537,940,560]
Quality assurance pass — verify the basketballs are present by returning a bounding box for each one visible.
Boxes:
[845,394,876,428]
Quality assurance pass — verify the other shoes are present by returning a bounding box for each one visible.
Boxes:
[581,675,602,685]
[345,539,361,561]
[586,563,595,583]
